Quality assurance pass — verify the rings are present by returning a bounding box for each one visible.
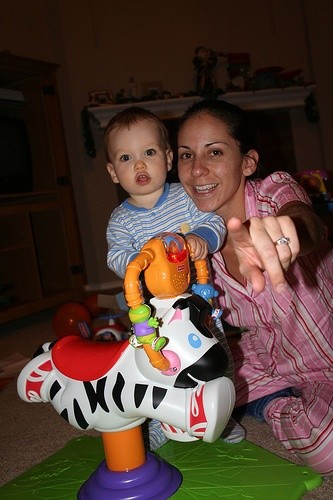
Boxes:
[273,236,290,246]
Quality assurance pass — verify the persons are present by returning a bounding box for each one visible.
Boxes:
[191,47,218,94]
[100,106,246,451]
[176,98,333,475]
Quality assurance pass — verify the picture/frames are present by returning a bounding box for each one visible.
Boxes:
[92,90,113,104]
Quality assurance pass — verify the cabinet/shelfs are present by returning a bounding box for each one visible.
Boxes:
[0,49,88,323]
